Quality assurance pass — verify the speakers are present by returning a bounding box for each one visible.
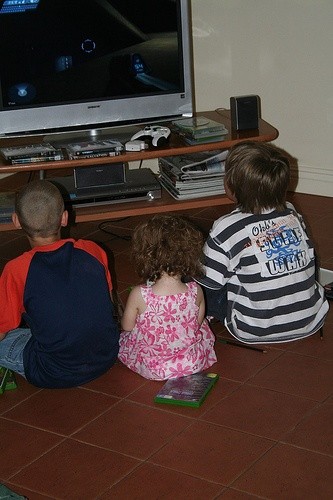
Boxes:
[231,95,262,131]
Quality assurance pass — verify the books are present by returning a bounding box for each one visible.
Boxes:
[154,373,218,408]
[158,150,229,200]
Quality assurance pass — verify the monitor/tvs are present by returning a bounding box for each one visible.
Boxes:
[0,1,194,140]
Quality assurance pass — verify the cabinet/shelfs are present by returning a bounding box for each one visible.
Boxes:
[0,110,279,232]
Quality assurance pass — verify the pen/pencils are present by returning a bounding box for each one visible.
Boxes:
[218,339,268,354]
[320,326,323,341]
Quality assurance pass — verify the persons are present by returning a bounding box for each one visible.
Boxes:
[118,213,216,380]
[0,180,119,388]
[193,141,330,345]
